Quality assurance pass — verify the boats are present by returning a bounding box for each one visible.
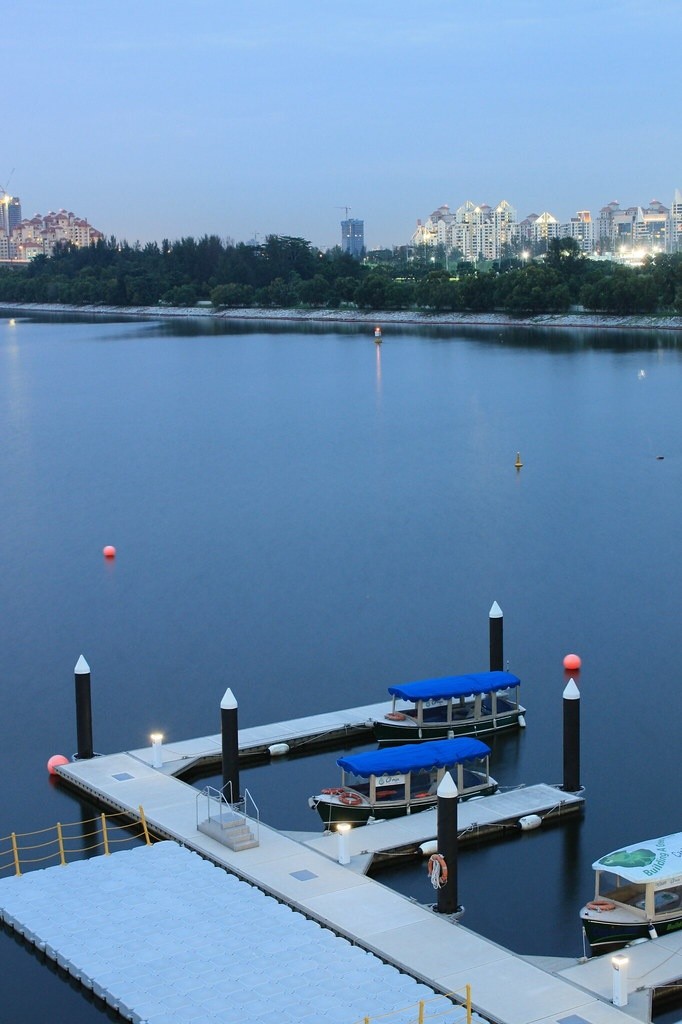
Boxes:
[374,670,527,746]
[580,831,682,955]
[308,736,498,831]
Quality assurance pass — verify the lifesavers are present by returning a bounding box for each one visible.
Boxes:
[321,787,344,794]
[586,900,616,910]
[339,792,362,805]
[384,712,405,720]
[427,853,448,882]
